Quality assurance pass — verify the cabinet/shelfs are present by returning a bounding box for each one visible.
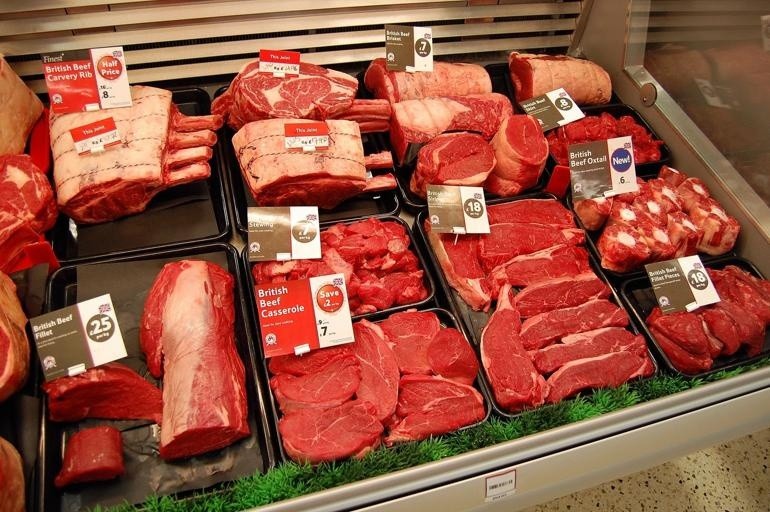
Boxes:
[1,0,768,512]
